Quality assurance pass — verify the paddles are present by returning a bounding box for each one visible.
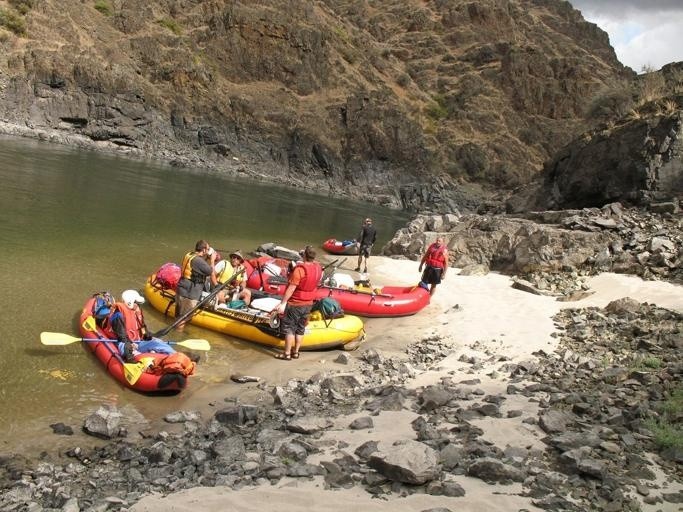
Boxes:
[40,332,210,350]
[153,268,246,337]
[267,276,392,297]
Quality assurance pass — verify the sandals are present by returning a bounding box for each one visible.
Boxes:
[274,351,300,360]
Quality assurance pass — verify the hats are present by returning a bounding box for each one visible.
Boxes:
[229,248,244,263]
[305,246,317,262]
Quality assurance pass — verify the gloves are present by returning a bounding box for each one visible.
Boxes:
[276,302,287,314]
[215,283,223,291]
[238,286,243,292]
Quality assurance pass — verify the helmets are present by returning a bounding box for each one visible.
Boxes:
[122,290,145,309]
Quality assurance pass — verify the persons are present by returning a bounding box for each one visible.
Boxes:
[210,248,252,310]
[419,236,448,304]
[106,289,177,365]
[355,218,377,273]
[274,246,322,360]
[173,239,219,334]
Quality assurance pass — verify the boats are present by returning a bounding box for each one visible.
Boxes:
[323,238,360,254]
[144,258,429,349]
[78,291,187,391]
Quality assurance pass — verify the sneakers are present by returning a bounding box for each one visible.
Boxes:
[355,268,368,272]
